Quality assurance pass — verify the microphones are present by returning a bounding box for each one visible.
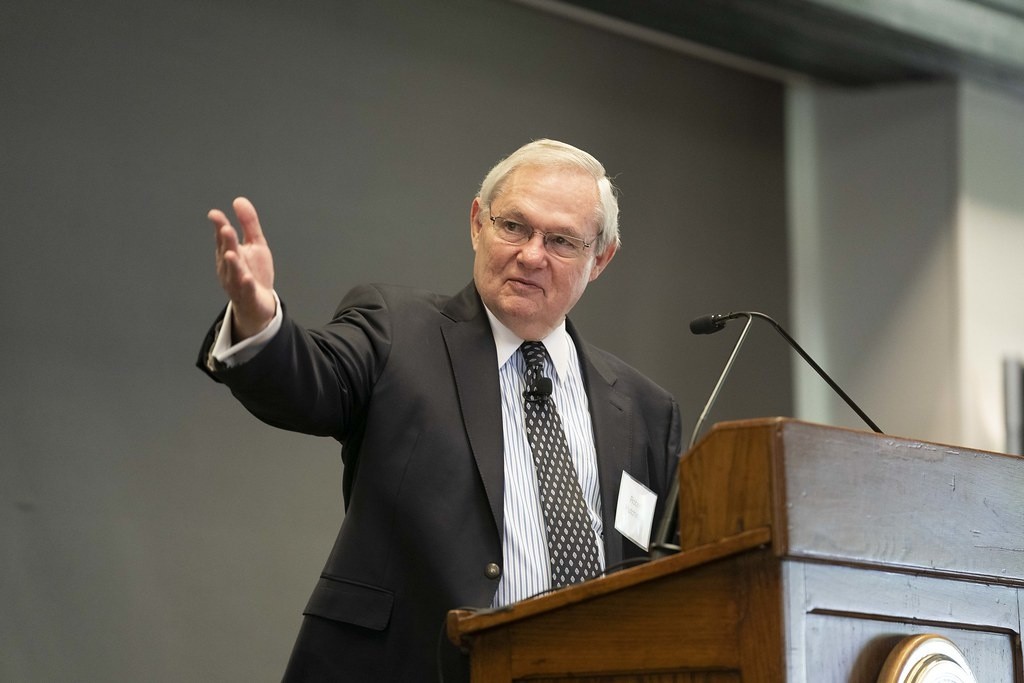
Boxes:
[652,311,885,563]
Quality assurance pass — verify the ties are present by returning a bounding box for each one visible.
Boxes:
[517,339,606,591]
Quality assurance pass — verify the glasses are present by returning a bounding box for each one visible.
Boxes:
[488,201,606,256]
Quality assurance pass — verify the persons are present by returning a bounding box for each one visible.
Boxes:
[194,136,686,683]
